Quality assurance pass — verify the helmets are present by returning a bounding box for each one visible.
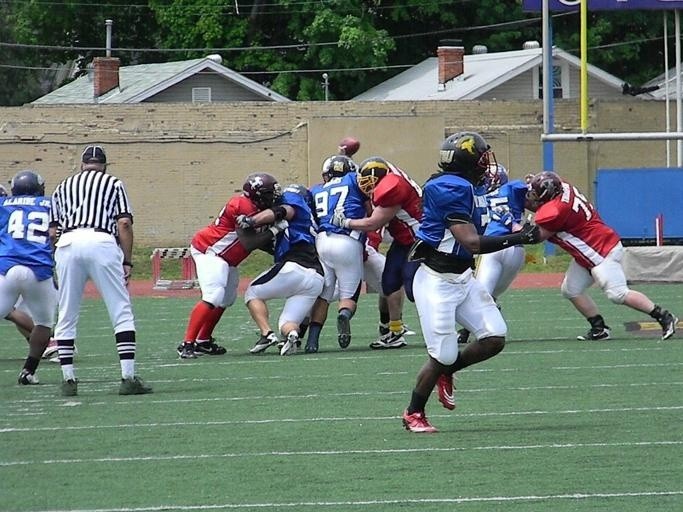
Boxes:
[439,131,499,193]
[486,163,507,191]
[525,171,563,208]
[10,171,43,196]
[356,157,389,196]
[285,183,313,207]
[321,154,355,182]
[242,172,282,207]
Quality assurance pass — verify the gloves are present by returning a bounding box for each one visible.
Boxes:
[269,220,288,236]
[521,222,540,244]
[235,214,255,228]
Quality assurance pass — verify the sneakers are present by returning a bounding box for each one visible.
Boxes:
[336,313,351,348]
[277,339,301,349]
[193,336,226,355]
[659,313,680,340]
[435,372,456,410]
[379,325,415,335]
[576,326,613,341]
[45,337,74,357]
[278,330,298,355]
[118,375,151,394]
[176,342,197,359]
[456,329,469,343]
[17,369,38,385]
[60,378,78,396]
[304,340,318,353]
[401,405,439,432]
[248,331,278,353]
[369,331,406,349]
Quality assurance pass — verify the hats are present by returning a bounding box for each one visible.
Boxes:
[80,145,105,164]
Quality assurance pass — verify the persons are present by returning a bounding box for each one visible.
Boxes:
[402,132,542,434]
[492,171,678,342]
[180,138,423,361]
[0,144,154,395]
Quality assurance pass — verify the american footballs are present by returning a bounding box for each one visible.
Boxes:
[340,137,360,157]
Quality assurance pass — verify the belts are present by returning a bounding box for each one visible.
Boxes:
[62,227,110,235]
